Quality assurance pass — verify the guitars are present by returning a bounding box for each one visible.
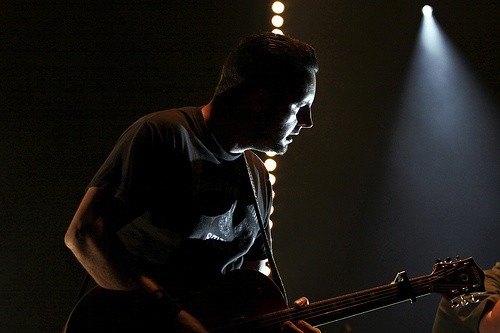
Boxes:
[63,254,486,333]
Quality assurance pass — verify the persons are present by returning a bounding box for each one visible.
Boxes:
[428,259,499,333]
[63,31,322,333]
